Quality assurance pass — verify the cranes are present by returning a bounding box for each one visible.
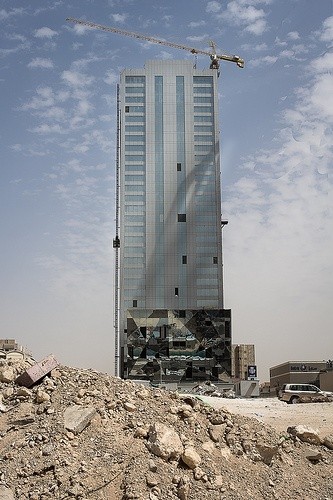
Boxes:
[62,15,245,80]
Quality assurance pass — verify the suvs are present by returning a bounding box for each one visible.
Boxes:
[277,383,333,404]
